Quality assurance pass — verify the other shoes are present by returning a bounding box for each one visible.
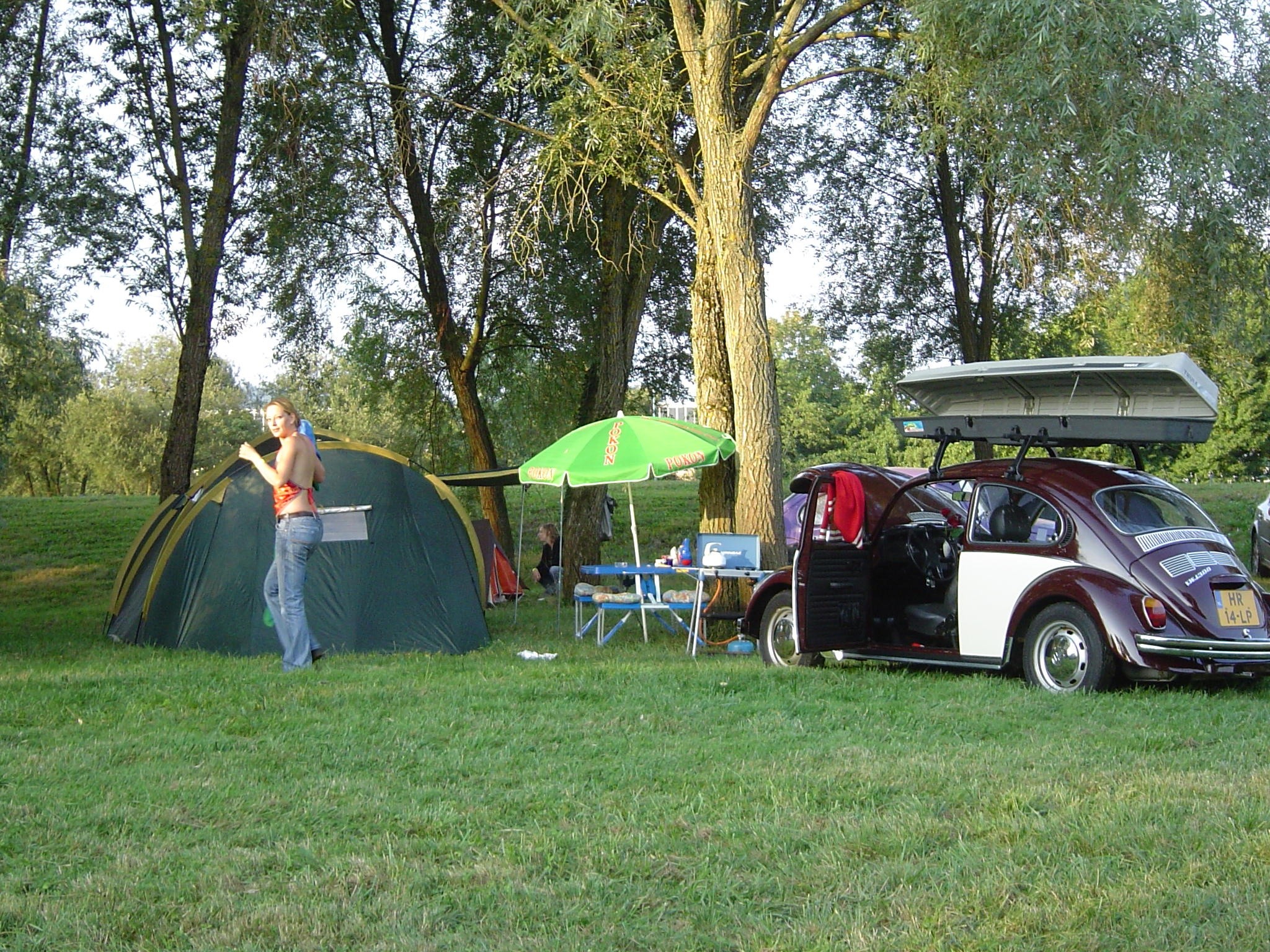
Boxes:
[311,648,324,665]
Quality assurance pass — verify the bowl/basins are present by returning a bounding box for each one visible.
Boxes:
[614,562,628,568]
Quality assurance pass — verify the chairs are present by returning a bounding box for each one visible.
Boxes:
[906,506,1030,637]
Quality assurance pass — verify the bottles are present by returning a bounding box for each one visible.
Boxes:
[679,537,692,568]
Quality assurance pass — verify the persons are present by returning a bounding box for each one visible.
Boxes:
[532,523,568,603]
[238,396,329,674]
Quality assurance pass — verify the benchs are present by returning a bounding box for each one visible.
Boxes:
[597,602,707,648]
[575,595,675,637]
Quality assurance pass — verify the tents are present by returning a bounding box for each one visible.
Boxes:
[469,519,534,608]
[99,428,583,660]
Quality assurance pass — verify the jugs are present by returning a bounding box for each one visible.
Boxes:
[703,541,727,569]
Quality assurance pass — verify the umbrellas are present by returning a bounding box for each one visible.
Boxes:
[517,410,737,643]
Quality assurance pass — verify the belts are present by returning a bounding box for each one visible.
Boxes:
[278,510,319,519]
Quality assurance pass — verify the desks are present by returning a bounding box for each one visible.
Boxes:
[581,564,697,575]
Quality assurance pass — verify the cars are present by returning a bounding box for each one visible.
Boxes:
[736,353,1270,692]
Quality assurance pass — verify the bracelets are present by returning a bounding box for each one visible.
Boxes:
[532,567,538,573]
[251,457,266,468]
